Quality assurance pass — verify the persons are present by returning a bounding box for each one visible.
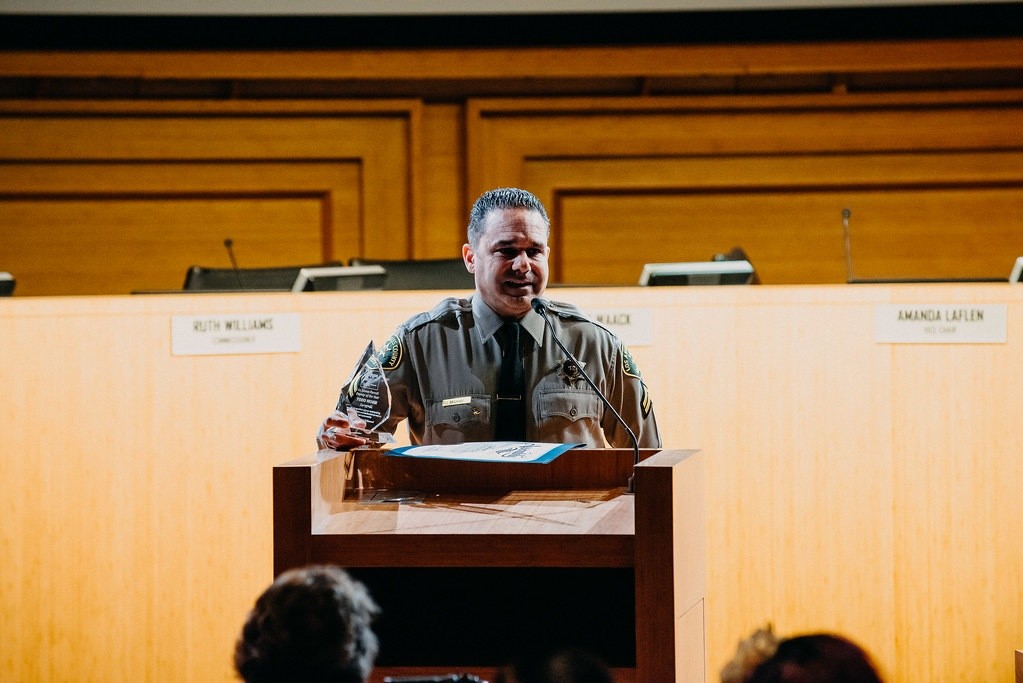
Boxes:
[316,187,663,452]
[720,624,881,683]
[233,565,379,683]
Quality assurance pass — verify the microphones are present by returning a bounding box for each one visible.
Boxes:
[530,298,639,494]
[224,239,247,290]
[841,209,852,283]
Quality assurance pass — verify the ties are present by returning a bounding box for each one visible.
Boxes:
[490,322,527,441]
